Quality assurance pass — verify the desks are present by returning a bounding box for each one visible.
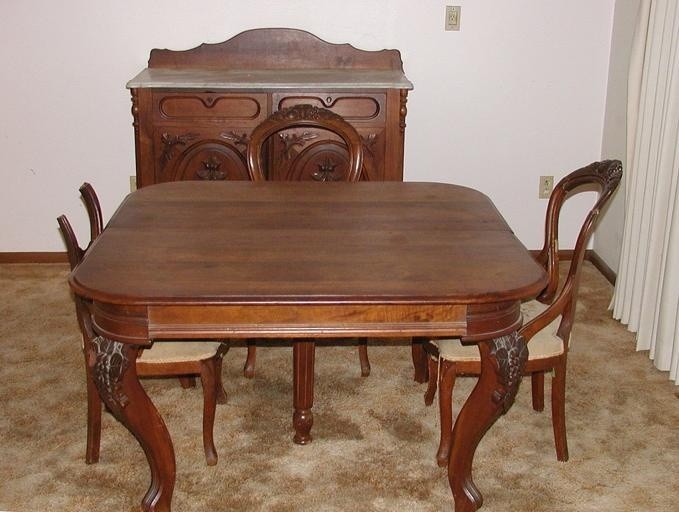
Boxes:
[67,179,552,512]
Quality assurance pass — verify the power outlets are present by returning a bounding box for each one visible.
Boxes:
[445,5,461,31]
[539,175,554,200]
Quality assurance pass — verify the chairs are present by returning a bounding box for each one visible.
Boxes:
[243,102,372,379]
[424,160,624,467]
[57,182,228,467]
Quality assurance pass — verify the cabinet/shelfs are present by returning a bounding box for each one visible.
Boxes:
[126,28,413,189]
